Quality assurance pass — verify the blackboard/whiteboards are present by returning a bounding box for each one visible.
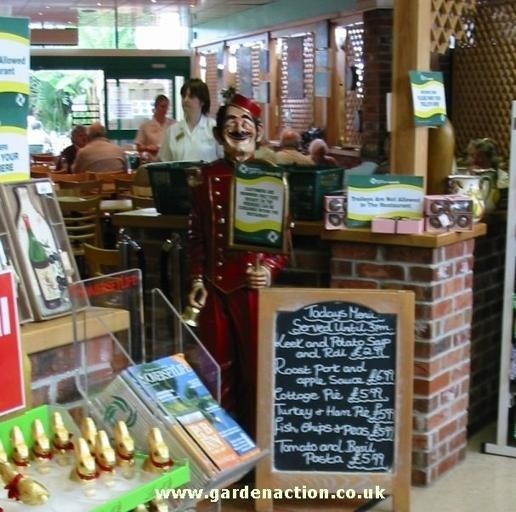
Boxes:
[258,288,414,511]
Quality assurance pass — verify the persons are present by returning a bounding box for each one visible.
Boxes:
[71,123,127,198]
[134,94,178,163]
[274,130,316,165]
[156,78,225,163]
[465,137,509,188]
[343,130,386,192]
[306,138,337,168]
[56,126,88,174]
[187,94,290,418]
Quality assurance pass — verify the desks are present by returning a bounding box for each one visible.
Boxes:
[112,207,189,242]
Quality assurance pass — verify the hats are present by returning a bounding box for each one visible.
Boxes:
[228,91,263,120]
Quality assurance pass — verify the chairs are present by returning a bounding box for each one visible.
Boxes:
[84,243,166,324]
[70,179,104,195]
[46,172,89,189]
[33,155,59,169]
[46,195,101,270]
[86,167,126,196]
[113,178,155,208]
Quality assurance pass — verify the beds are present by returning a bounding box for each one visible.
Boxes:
[58,197,133,214]
